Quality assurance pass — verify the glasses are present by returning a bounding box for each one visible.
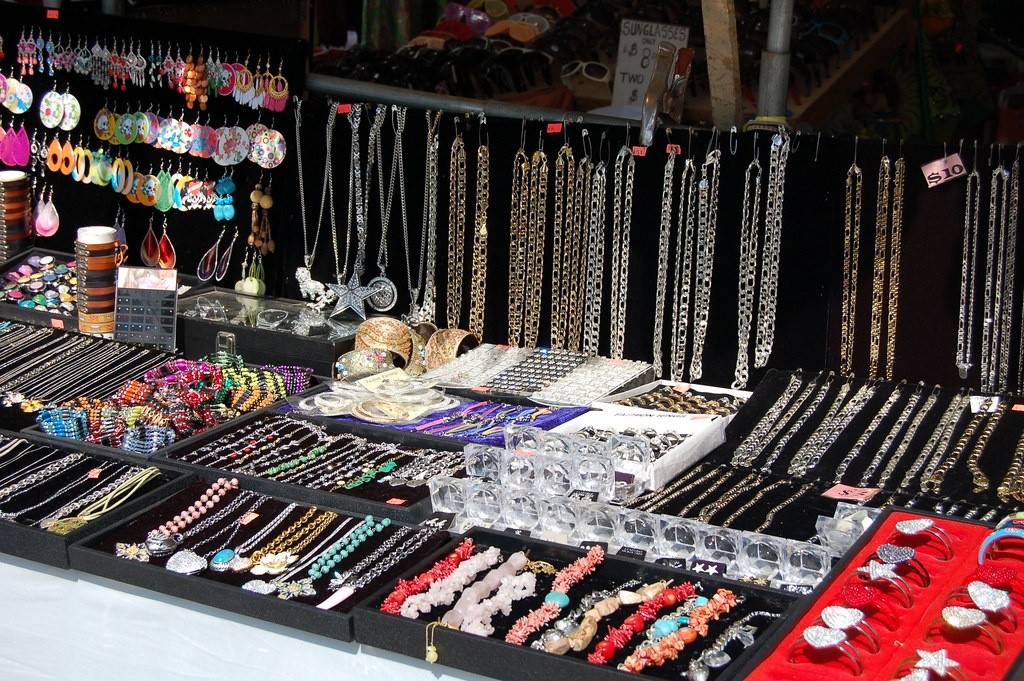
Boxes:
[309,1,908,99]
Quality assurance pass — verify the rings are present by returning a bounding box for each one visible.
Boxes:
[789,517,1024,680]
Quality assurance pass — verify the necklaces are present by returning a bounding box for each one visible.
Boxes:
[0,93,792,681]
[731,132,1024,536]
